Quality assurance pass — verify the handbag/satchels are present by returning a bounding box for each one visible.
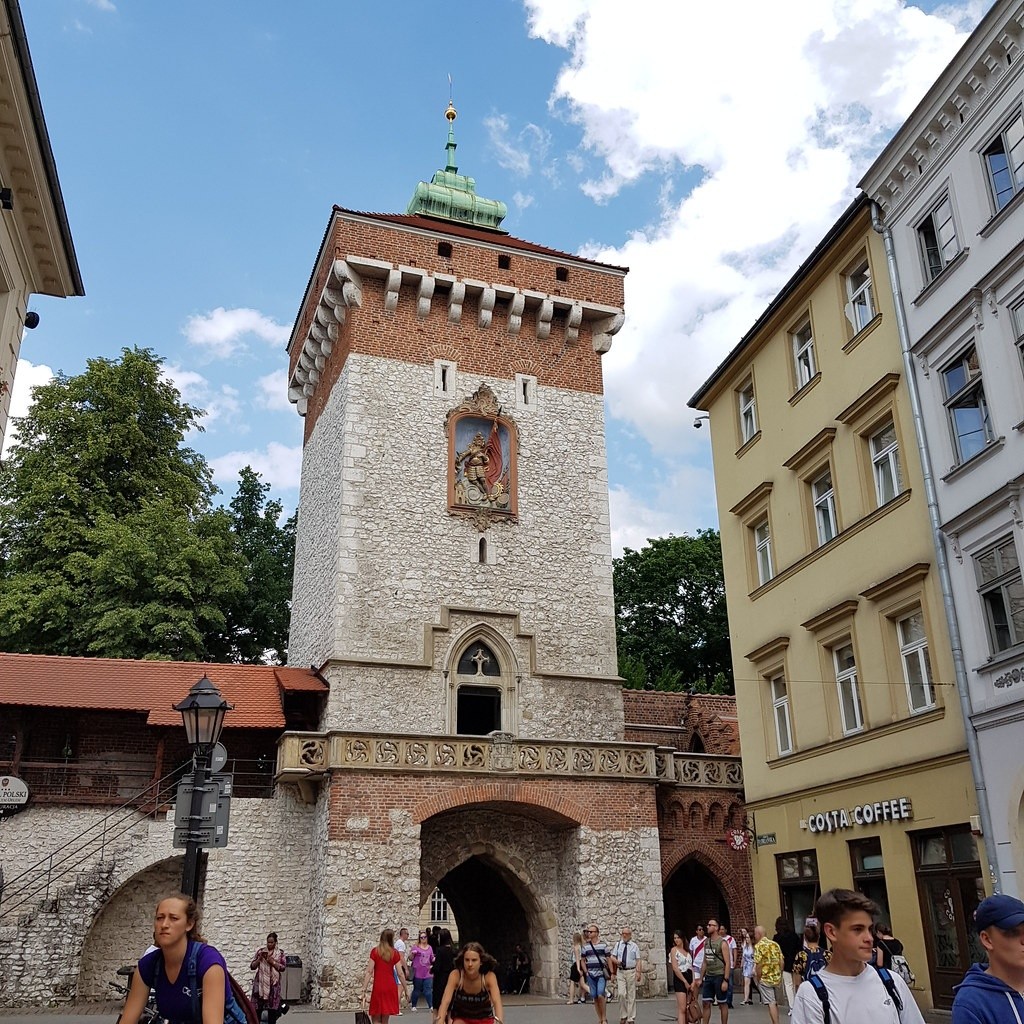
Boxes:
[401,957,415,981]
[602,966,611,980]
[686,988,702,1024]
[355,998,371,1024]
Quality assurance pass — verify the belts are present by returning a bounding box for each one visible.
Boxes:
[619,967,635,970]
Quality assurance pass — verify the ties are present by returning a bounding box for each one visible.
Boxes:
[621,942,627,968]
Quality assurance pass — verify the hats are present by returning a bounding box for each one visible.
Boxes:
[976,895,1024,935]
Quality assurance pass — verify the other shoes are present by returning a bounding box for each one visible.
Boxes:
[620,1017,627,1024]
[431,1006,434,1012]
[410,1006,417,1012]
[739,1000,753,1005]
[566,1001,574,1004]
[574,999,586,1004]
[606,993,614,1003]
[629,1018,634,1024]
[712,1001,718,1005]
[397,1011,403,1015]
[728,1003,734,1009]
[787,1009,792,1016]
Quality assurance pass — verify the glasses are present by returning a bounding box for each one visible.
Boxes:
[587,930,596,933]
[697,928,704,931]
[420,936,427,939]
[706,923,718,927]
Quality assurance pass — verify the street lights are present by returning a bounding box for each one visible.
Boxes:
[170,669,235,898]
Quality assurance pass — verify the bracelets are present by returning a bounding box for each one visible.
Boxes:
[585,971,588,973]
[724,978,729,983]
[272,961,275,966]
[362,989,366,992]
[611,972,614,974]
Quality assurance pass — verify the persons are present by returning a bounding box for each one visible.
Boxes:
[394,926,528,1016]
[668,917,904,1024]
[249,932,286,1024]
[574,930,614,1004]
[611,928,642,1024]
[142,932,162,999]
[456,433,496,502]
[582,926,614,1024]
[951,894,1024,1024]
[436,942,504,1024]
[791,888,926,1024]
[117,893,249,1024]
[361,929,409,1024]
[564,933,590,1005]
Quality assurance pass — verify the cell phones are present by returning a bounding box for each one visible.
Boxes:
[262,952,268,957]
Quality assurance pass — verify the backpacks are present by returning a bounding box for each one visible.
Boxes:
[153,941,259,1024]
[880,939,915,984]
[804,947,827,981]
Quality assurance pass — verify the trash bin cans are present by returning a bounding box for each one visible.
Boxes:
[281,956,303,1004]
